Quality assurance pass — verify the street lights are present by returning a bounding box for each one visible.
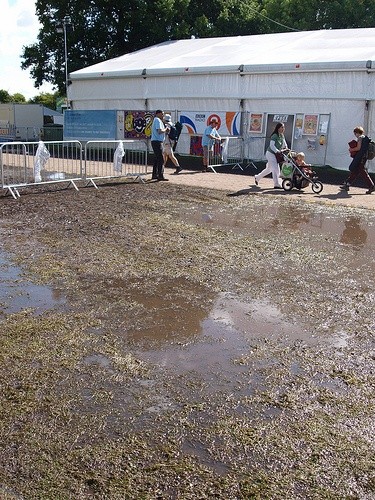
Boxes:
[55,22,73,86]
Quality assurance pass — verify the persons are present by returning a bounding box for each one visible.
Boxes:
[162,114,183,174]
[201,119,222,172]
[295,152,313,174]
[339,127,375,194]
[255,122,288,189]
[150,110,172,181]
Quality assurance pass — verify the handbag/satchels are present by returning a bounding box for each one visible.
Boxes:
[276,153,284,163]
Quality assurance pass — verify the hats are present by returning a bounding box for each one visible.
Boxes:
[209,119,218,125]
[163,114,171,120]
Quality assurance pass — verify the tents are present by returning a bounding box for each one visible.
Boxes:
[66,28,374,175]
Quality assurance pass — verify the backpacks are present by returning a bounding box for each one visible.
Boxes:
[366,138,375,161]
[166,124,178,141]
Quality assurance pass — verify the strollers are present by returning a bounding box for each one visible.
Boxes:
[281,149,323,193]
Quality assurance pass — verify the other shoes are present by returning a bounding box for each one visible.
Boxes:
[366,186,375,195]
[255,175,258,185]
[152,173,169,181]
[174,166,182,173]
[340,184,349,191]
[202,167,213,173]
[274,186,283,189]
